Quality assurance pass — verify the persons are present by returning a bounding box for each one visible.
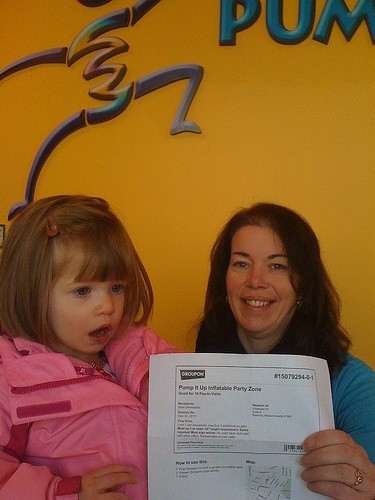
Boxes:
[191,199,375,500]
[0,193,193,500]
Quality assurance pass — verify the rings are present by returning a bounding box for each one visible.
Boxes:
[348,468,363,488]
